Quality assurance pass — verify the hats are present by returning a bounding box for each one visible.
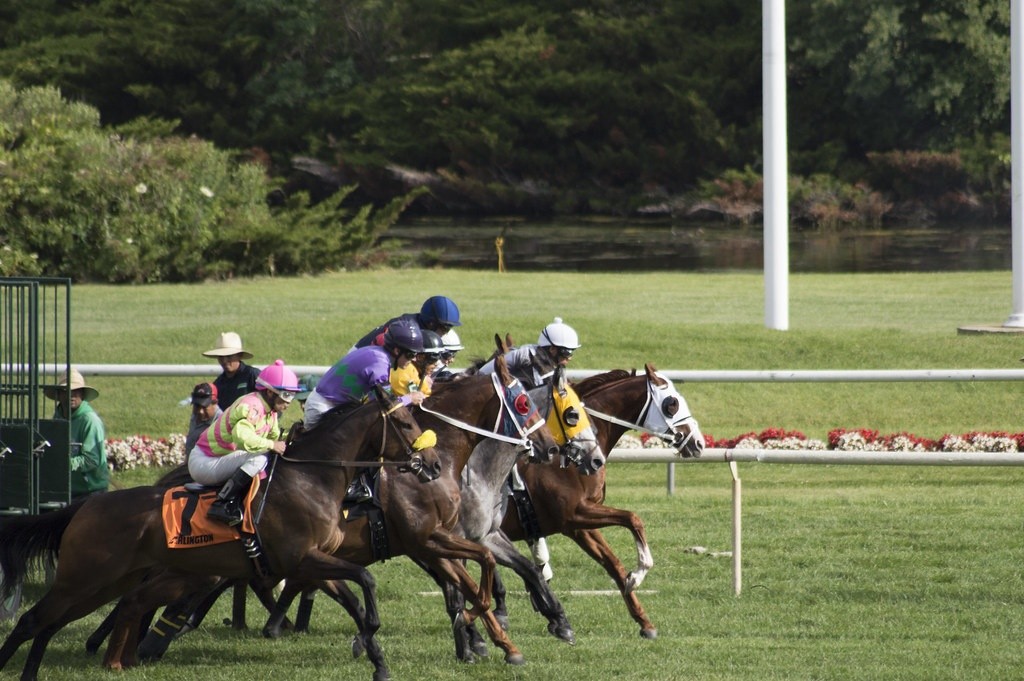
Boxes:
[190,383,218,407]
[43,369,99,402]
[202,332,254,359]
[296,374,322,400]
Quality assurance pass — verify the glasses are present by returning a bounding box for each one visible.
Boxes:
[192,391,218,400]
[424,353,442,365]
[279,390,295,404]
[558,346,573,359]
[441,324,450,334]
[405,350,419,359]
[442,351,454,361]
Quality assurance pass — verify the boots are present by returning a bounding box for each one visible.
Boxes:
[207,468,253,528]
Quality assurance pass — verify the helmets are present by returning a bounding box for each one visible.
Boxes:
[441,329,465,350]
[255,359,301,391]
[420,329,448,354]
[538,317,581,349]
[384,316,423,353]
[420,296,461,326]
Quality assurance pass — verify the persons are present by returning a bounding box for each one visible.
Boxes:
[389,329,449,399]
[202,331,262,412]
[44,369,109,517]
[188,359,301,522]
[304,321,424,499]
[347,296,462,355]
[185,382,224,459]
[474,317,581,390]
[295,374,322,412]
[433,328,464,371]
